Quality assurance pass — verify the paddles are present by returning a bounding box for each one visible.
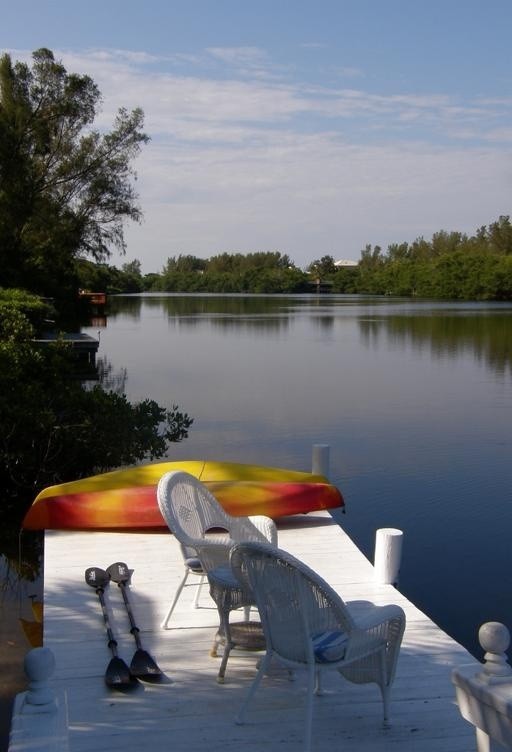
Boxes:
[85,568,138,690]
[106,563,162,679]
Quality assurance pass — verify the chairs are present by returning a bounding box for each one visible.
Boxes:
[229,541,406,751]
[157,470,277,630]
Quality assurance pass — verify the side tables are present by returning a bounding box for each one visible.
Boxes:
[208,566,298,683]
